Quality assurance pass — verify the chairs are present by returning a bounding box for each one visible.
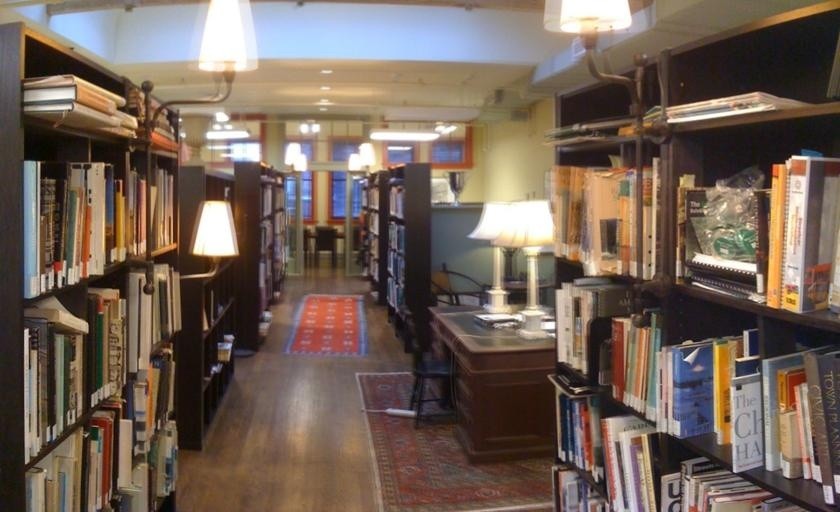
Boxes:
[409,317,451,429]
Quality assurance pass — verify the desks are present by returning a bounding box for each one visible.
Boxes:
[424,301,556,462]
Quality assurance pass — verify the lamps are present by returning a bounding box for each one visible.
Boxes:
[139,0,263,299]
[459,199,558,340]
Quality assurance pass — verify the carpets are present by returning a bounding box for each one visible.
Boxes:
[356,368,553,510]
[284,290,372,359]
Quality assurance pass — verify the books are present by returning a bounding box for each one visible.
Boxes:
[471,309,521,329]
[256,173,288,340]
[203,299,237,381]
[357,172,408,313]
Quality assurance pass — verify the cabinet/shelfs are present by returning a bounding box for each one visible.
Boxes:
[356,160,431,353]
[178,157,287,452]
[538,1,840,512]
[3,20,184,512]
